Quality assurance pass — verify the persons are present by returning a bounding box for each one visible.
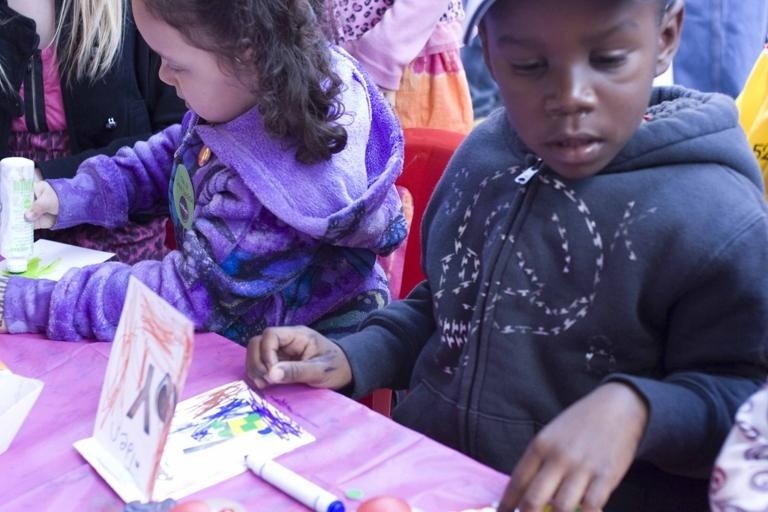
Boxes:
[246,0,767,512]
[0,1,185,264]
[0,0,409,348]
[673,0,768,100]
[320,2,474,135]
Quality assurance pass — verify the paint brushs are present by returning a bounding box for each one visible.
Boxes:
[243,450,345,512]
